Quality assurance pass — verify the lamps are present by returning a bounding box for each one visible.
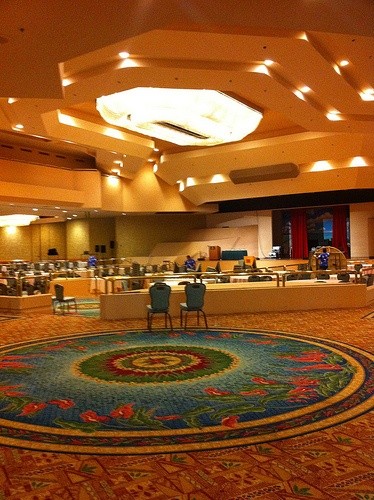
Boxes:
[96,87,263,146]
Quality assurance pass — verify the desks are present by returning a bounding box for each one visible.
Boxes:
[99,284,374,321]
[48,276,150,299]
[0,293,53,313]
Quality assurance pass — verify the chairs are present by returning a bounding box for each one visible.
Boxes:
[179,282,208,331]
[145,283,174,333]
[0,260,374,315]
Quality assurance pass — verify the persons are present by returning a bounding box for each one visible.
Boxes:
[314,248,330,271]
[184,255,195,272]
[87,254,96,268]
